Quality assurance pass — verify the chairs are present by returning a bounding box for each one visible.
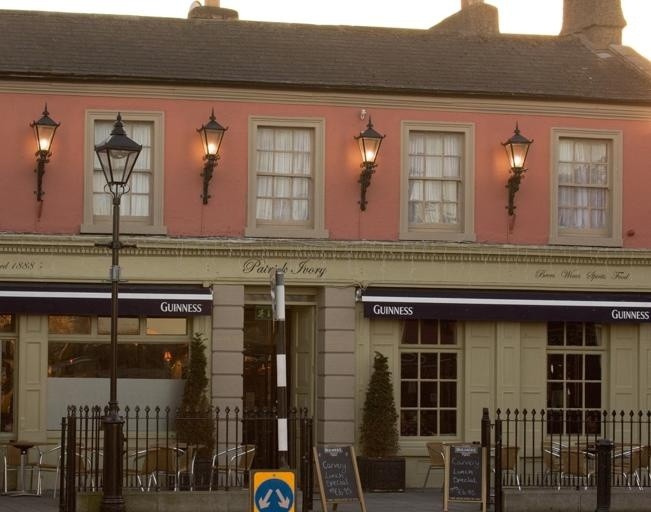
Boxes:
[422,441,648,492]
[0,442,258,500]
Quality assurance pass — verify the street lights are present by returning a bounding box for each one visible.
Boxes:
[95,112,142,512]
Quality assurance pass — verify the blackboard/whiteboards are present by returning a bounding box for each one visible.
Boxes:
[313,444,363,503]
[445,445,487,503]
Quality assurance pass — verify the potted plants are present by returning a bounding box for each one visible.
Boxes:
[168,331,216,489]
[355,353,405,493]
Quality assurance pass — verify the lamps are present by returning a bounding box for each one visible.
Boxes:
[196,107,230,205]
[500,120,535,216]
[30,102,61,201]
[353,115,387,211]
